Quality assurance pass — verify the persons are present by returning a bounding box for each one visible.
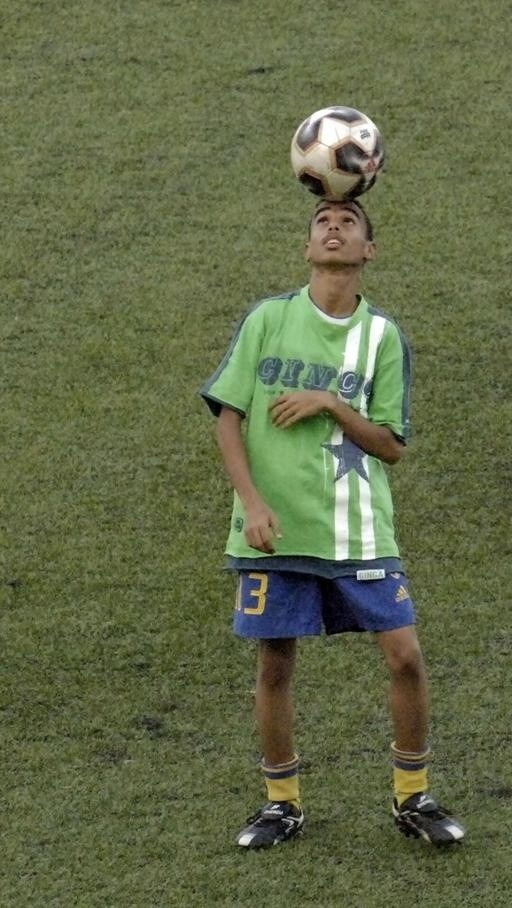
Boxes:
[200,198,468,849]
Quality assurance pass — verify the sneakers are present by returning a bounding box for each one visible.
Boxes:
[392,792,465,849]
[234,801,307,851]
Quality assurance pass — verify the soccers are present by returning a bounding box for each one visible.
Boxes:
[291,105,383,203]
